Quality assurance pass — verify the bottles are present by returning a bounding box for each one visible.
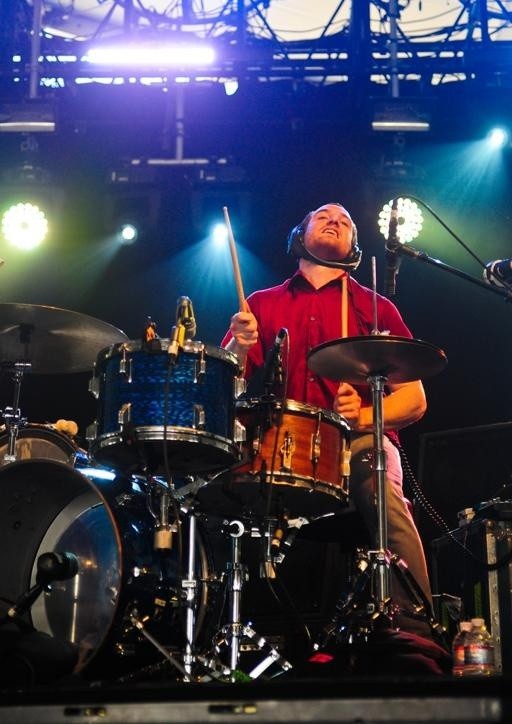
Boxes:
[451,622,471,676]
[463,617,495,676]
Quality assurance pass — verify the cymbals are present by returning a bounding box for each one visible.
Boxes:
[1,302,130,382]
[305,335,448,385]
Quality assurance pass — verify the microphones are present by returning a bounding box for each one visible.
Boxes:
[175,295,197,340]
[457,496,503,518]
[261,328,287,375]
[384,199,398,297]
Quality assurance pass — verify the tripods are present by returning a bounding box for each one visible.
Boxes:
[144,495,296,681]
[316,386,451,668]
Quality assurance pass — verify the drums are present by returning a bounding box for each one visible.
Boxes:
[219,398,352,508]
[1,459,227,698]
[87,338,247,470]
[0,424,92,470]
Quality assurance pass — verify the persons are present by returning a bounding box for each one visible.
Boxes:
[221,203,435,639]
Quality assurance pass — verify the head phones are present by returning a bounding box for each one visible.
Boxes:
[287,224,362,270]
